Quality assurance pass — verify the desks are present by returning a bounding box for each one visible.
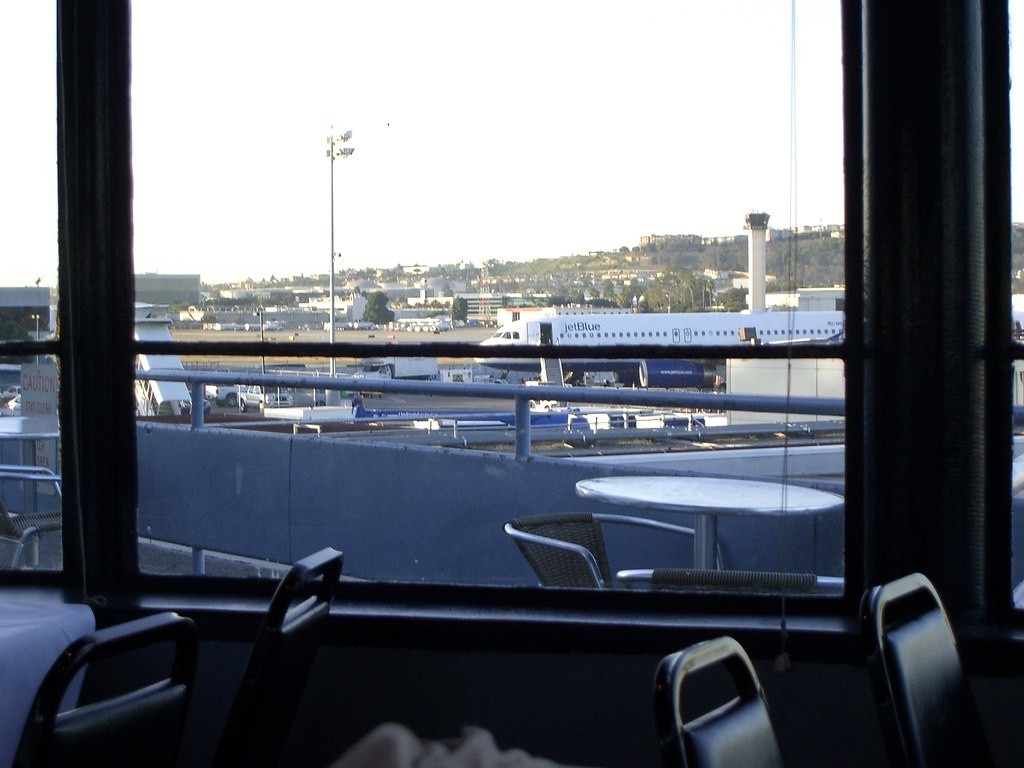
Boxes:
[0,601,96,768]
[573,474,845,570]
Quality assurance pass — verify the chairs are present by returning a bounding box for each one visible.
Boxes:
[200,544,344,768]
[859,571,994,768]
[503,510,847,595]
[655,635,788,768]
[11,613,200,768]
[0,463,63,570]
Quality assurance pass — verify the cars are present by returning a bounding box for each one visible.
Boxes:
[1,385,21,411]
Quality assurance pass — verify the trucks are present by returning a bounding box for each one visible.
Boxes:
[324,321,378,330]
[395,320,451,332]
[453,320,465,328]
[202,322,283,331]
[350,355,439,379]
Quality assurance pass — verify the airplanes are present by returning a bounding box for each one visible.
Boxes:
[471,296,1024,387]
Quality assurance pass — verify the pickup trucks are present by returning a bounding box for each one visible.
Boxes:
[237,385,293,413]
[204,384,255,408]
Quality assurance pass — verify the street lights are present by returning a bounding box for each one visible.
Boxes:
[31,314,41,364]
[325,128,355,378]
